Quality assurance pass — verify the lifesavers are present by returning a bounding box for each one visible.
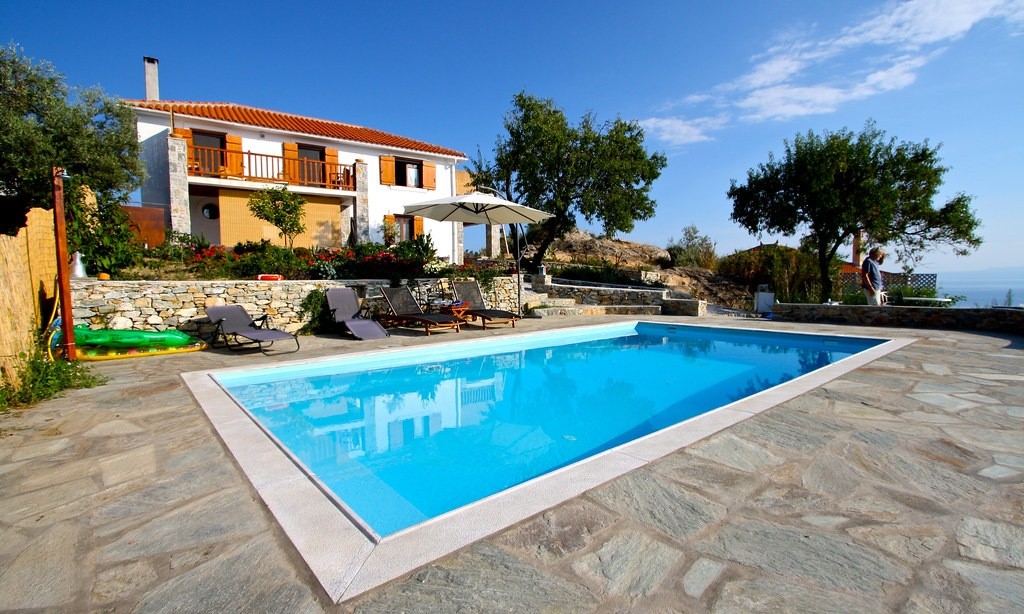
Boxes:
[254,273,283,281]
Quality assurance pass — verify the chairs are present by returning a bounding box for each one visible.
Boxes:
[205,304,300,357]
[452,280,521,329]
[380,285,466,336]
[323,285,390,339]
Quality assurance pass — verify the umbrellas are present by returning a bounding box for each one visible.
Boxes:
[402,189,557,320]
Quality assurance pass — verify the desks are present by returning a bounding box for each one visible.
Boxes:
[440,302,471,334]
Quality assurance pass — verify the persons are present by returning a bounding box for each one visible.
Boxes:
[860,247,887,305]
